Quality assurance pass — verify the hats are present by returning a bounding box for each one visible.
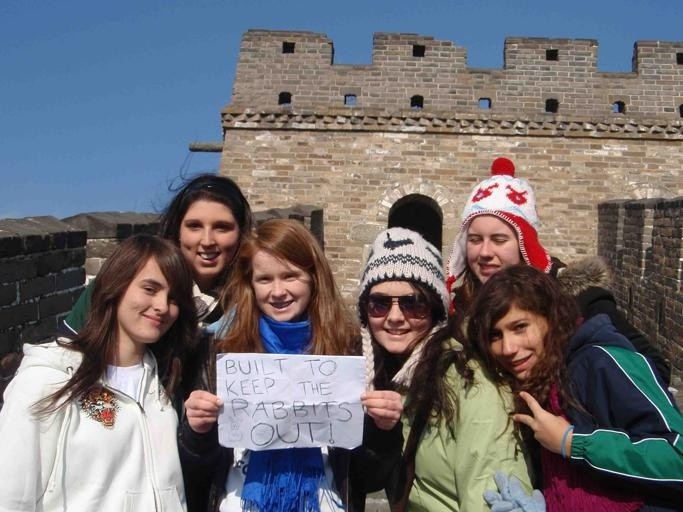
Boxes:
[445,157,552,282]
[355,226,451,332]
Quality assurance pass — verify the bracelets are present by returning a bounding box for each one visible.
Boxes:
[560,424,575,458]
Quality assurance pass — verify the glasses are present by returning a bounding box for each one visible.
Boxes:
[366,295,432,319]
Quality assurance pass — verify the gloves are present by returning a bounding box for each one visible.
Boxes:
[482,470,546,512]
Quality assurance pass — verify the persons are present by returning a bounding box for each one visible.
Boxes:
[471,263,682,512]
[354,227,539,511]
[3,233,199,511]
[443,160,671,418]
[169,216,408,512]
[138,173,254,408]
